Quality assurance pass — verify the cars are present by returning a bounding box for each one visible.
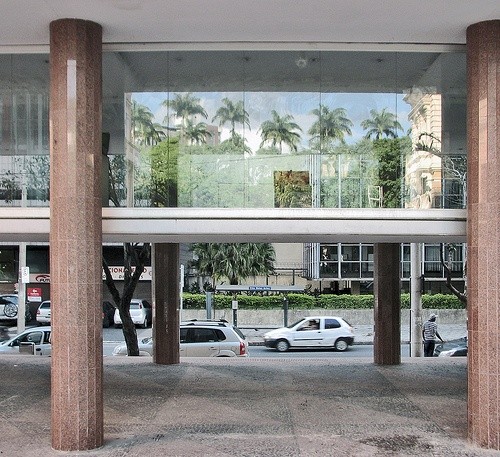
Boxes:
[434,345,468,357]
[263,316,355,351]
[36,300,50,326]
[102,301,114,327]
[114,297,152,327]
[0,326,51,354]
[113,318,250,359]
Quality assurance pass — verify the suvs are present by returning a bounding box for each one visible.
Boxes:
[0,294,31,325]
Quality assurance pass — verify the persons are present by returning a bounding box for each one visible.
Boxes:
[422,313,445,357]
[303,319,319,330]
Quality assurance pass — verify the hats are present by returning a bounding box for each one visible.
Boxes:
[427,313,439,321]
